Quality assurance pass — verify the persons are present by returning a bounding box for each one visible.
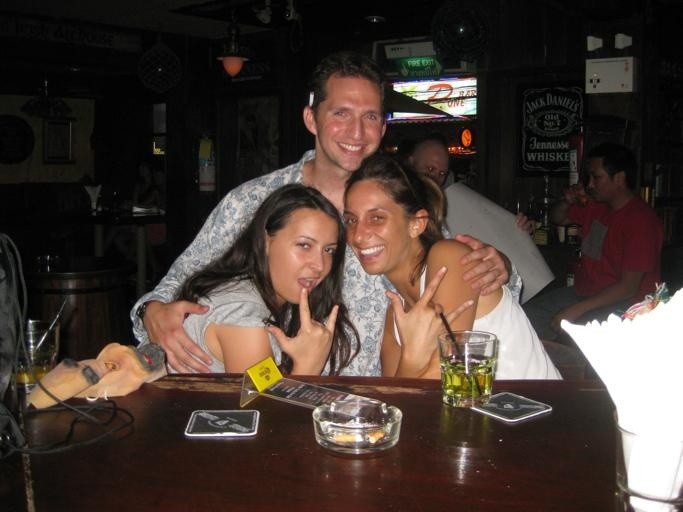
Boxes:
[524,139,665,346]
[129,50,525,378]
[406,138,535,238]
[342,149,565,383]
[135,182,360,376]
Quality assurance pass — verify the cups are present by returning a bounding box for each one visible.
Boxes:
[575,186,597,208]
[438,331,500,408]
[11,319,61,397]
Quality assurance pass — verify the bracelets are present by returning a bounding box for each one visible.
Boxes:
[135,300,147,319]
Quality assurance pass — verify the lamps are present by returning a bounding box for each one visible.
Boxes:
[216,8,253,77]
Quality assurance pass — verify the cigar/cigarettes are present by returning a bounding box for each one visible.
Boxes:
[367,430,385,443]
[334,432,364,442]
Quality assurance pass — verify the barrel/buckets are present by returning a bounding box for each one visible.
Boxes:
[35,268,129,363]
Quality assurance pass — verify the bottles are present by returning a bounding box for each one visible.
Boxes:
[196,129,215,192]
